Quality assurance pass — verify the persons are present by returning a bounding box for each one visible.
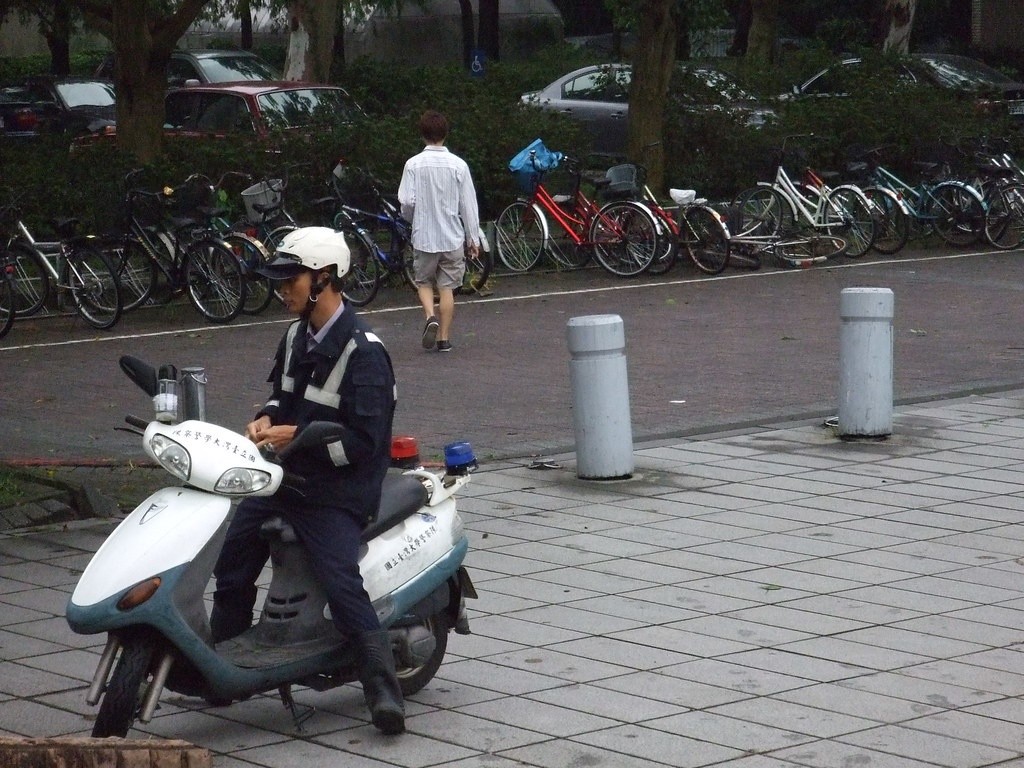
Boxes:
[209,227,406,733]
[397,110,481,352]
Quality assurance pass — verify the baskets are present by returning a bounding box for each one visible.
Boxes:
[607,165,647,195]
[747,145,781,167]
[241,178,281,223]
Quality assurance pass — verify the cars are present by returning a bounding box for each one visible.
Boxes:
[520,62,779,135]
[69,81,378,164]
[0,77,180,139]
[167,48,283,83]
[772,51,1024,133]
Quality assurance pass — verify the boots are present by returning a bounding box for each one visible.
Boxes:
[352,629,405,735]
[210,601,253,702]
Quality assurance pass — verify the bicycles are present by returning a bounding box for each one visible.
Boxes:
[1,139,1023,334]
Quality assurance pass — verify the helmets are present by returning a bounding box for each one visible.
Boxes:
[254,226,350,279]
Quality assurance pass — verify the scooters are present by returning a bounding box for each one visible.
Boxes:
[64,354,478,739]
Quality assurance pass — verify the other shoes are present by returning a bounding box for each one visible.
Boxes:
[422,317,439,349]
[438,340,452,352]
